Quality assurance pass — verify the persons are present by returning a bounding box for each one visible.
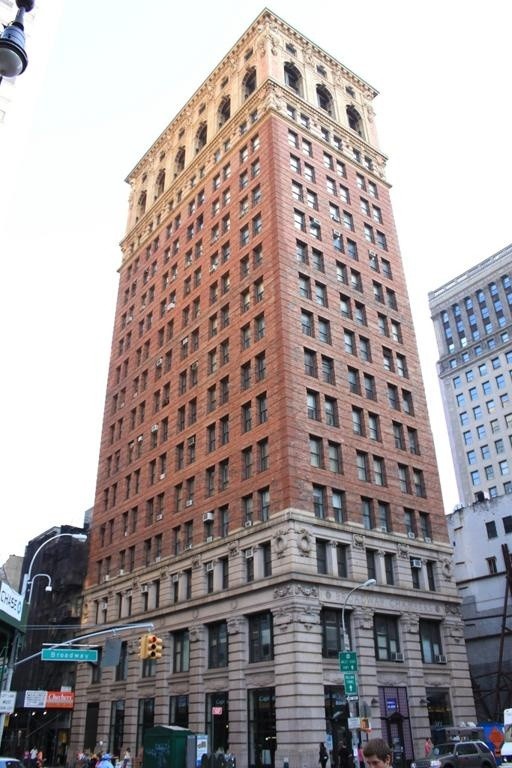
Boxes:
[22,745,43,768]
[77,747,131,768]
[318,737,431,768]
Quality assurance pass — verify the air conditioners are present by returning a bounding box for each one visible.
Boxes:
[426,538,431,543]
[369,251,375,257]
[312,220,320,225]
[210,266,216,272]
[204,514,212,520]
[408,532,415,539]
[157,359,163,366]
[160,474,166,479]
[186,500,192,507]
[391,653,405,662]
[246,522,252,527]
[411,559,421,568]
[435,654,447,664]
[333,215,340,221]
[151,425,158,433]
[103,604,107,609]
[157,515,162,521]
[189,437,197,446]
[333,231,340,237]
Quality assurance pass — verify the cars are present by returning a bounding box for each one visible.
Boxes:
[411,741,497,767]
[0,757,24,767]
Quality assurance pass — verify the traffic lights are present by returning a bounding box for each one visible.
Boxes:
[137,635,162,660]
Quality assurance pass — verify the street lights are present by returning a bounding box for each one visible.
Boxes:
[341,578,377,740]
[1,534,89,753]
[0,1,35,77]
